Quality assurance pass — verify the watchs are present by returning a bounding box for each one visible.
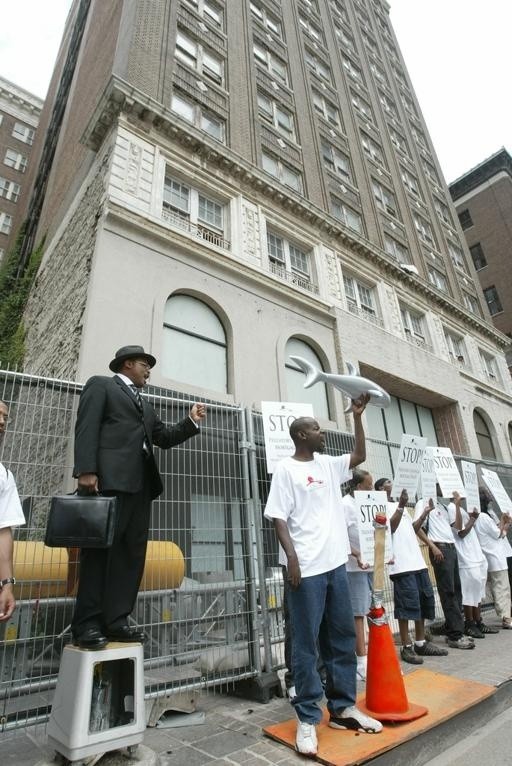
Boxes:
[396,506,405,514]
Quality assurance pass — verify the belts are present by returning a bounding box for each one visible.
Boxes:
[436,543,454,547]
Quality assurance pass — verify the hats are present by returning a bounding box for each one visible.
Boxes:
[109,345,156,373]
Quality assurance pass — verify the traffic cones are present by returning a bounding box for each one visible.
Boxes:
[357,608,429,722]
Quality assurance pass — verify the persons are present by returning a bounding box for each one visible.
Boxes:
[69,345,207,650]
[413,483,476,648]
[373,477,450,665]
[445,496,500,638]
[478,487,512,590]
[473,492,511,629]
[342,469,397,681]
[262,389,384,757]
[0,400,26,624]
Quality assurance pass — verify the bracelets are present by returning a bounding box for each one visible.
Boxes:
[0,574,16,588]
[471,516,476,520]
[420,515,426,520]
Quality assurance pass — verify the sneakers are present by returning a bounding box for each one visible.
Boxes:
[400,619,512,664]
[287,686,297,703]
[328,706,383,733]
[356,666,367,681]
[295,720,318,755]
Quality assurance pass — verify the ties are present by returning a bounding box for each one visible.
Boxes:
[131,384,150,449]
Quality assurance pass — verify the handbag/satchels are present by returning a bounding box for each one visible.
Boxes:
[45,489,117,551]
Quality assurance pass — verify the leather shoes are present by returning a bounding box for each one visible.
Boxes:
[73,629,108,651]
[103,626,145,642]
